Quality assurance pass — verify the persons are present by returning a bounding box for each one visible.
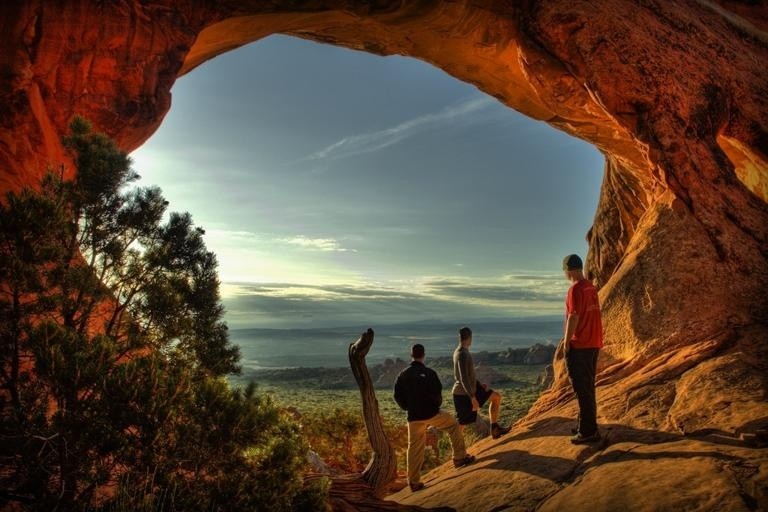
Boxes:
[452,326,512,439]
[555,254,603,444]
[393,343,475,491]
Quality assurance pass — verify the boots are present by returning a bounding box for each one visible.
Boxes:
[489,422,512,440]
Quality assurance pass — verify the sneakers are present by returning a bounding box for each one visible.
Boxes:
[570,422,601,445]
[453,455,476,469]
[409,481,424,492]
